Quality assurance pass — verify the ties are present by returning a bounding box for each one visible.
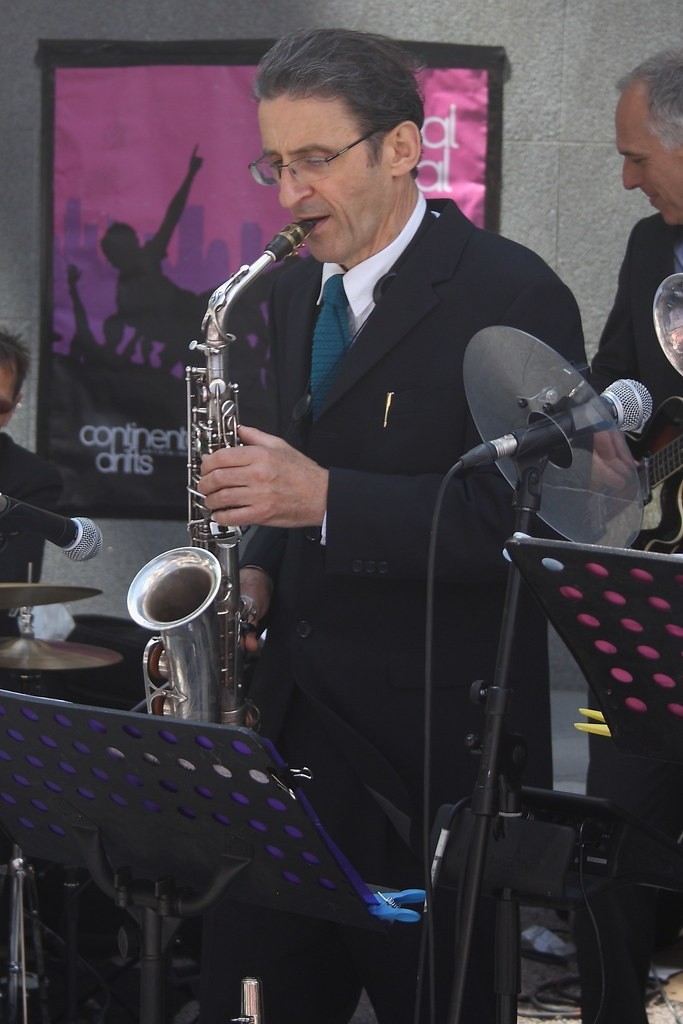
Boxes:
[310,274,350,417]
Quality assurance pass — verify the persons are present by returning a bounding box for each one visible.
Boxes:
[0,332,62,648]
[531,45,683,1013]
[159,31,587,1024]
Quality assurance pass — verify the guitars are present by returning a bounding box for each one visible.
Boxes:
[588,394,683,553]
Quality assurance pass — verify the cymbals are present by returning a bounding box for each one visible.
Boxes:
[1,581,102,612]
[0,632,123,672]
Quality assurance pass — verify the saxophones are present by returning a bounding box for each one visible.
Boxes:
[124,210,332,730]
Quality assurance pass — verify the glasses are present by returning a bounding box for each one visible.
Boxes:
[0,400,16,414]
[247,127,383,187]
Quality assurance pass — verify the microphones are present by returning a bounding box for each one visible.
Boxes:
[0,494,104,563]
[458,378,654,469]
